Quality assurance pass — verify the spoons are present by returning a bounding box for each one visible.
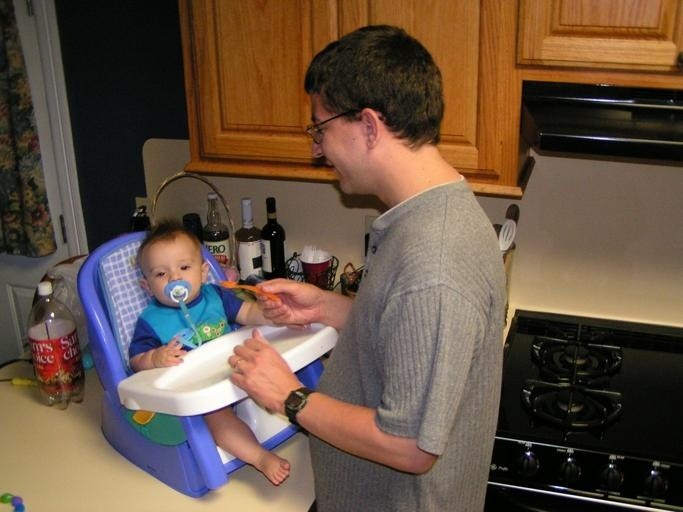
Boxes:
[217,278,279,304]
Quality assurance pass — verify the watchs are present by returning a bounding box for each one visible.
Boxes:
[284,386,315,428]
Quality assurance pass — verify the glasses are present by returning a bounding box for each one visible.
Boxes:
[303,107,385,145]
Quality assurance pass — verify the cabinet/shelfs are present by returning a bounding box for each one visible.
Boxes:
[177,0,524,200]
[516,0,683,74]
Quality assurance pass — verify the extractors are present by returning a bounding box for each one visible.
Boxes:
[521,81,682,169]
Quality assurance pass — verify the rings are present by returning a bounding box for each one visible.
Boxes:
[233,358,243,369]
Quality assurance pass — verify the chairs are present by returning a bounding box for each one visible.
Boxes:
[77,228,340,497]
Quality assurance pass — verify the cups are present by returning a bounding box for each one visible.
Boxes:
[300,251,336,290]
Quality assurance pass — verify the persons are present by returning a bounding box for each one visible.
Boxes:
[125,217,310,485]
[219,24,508,511]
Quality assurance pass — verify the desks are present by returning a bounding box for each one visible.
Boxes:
[0,348,317,512]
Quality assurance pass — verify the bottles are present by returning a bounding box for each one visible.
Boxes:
[24,280,85,412]
[257,197,291,282]
[234,196,264,287]
[199,190,231,273]
[181,213,204,246]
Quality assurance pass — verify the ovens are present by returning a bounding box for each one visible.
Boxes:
[483,477,682,512]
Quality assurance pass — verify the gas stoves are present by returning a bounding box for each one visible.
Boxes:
[476,307,682,509]
